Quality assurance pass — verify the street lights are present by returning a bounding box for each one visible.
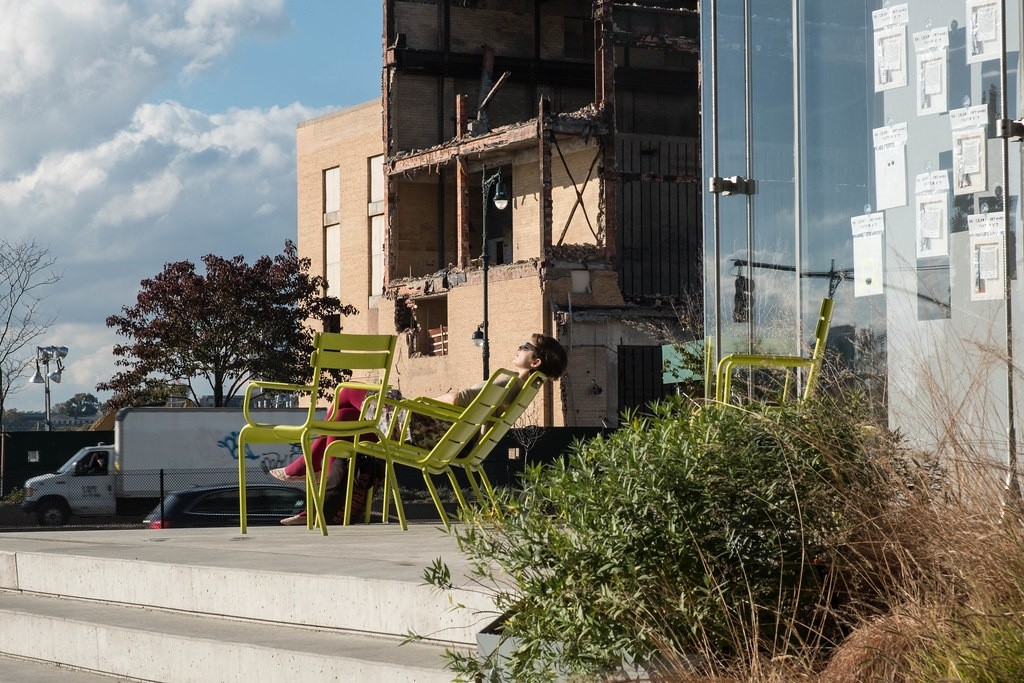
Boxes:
[471,162,509,389]
[29,346,69,431]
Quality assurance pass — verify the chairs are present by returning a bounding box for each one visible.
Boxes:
[711,293,835,420]
[235,333,414,537]
[357,371,551,522]
[316,367,521,529]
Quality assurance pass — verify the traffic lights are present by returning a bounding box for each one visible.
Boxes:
[731,274,757,324]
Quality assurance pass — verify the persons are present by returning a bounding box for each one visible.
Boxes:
[269,332,569,527]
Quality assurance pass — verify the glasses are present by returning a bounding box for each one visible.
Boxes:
[517,342,536,351]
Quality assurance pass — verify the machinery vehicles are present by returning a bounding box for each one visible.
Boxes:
[87,398,195,432]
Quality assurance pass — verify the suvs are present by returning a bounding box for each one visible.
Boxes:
[142,483,338,530]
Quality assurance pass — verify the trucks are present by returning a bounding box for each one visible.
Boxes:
[22,406,326,530]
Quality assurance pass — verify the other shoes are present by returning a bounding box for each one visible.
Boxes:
[279,514,307,525]
[269,466,329,483]
